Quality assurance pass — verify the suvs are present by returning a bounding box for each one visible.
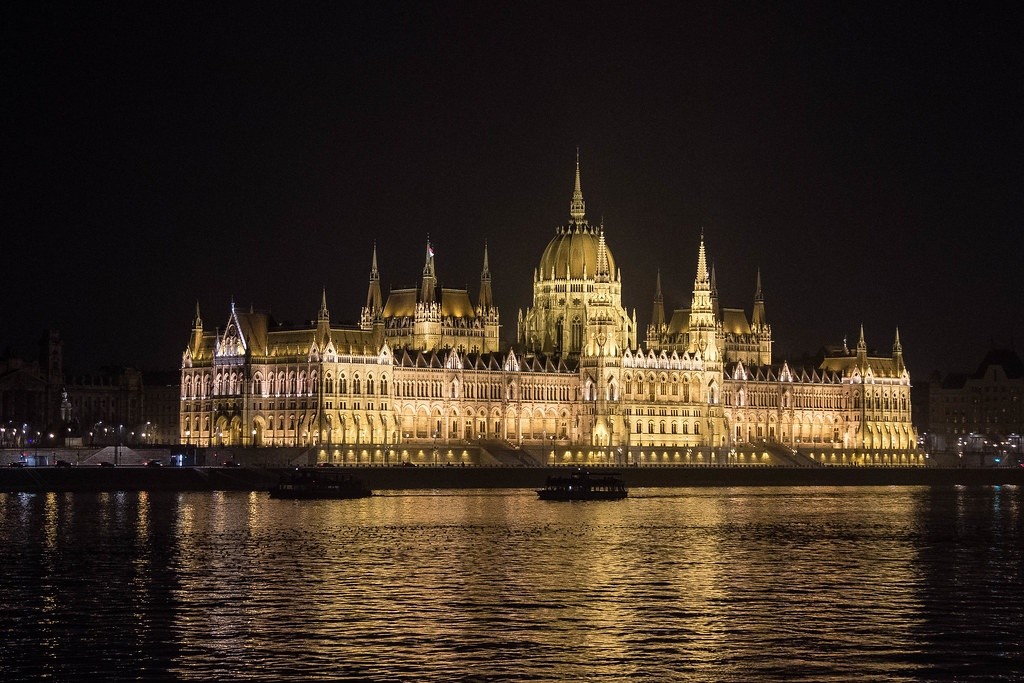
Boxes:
[54,459,71,468]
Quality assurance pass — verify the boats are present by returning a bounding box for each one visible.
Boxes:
[267,465,372,501]
[533,467,628,502]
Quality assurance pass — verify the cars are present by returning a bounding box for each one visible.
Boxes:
[9,462,29,467]
[96,461,115,468]
[144,460,166,467]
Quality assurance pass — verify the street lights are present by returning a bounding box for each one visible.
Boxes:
[68,428,72,449]
[146,421,151,444]
[119,424,123,466]
[251,429,257,446]
[35,432,41,457]
[184,429,190,444]
